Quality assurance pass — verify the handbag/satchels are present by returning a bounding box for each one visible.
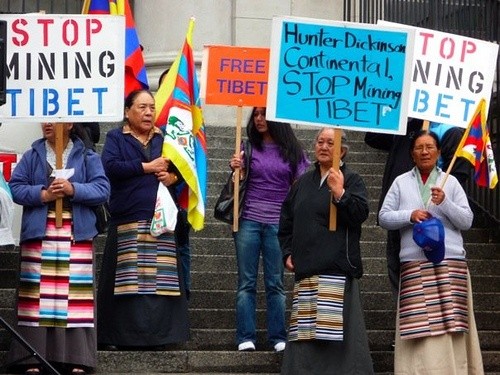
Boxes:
[81,149,111,233]
[214,138,252,223]
[150,182,178,237]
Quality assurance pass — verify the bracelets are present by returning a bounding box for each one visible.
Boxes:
[174,175,178,182]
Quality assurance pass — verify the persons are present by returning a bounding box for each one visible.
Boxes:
[8,122,110,375]
[379,130,486,375]
[69,122,106,237]
[158,70,192,300]
[278,126,377,375]
[230,107,312,352]
[95,89,191,349]
[363,118,474,347]
[150,22,206,231]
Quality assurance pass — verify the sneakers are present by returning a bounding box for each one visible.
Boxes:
[238,341,257,351]
[274,340,286,353]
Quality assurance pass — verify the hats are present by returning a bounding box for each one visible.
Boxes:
[412,217,445,263]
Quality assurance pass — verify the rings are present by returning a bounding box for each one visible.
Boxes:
[60,184,64,188]
[434,195,437,198]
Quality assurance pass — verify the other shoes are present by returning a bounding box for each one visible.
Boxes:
[153,348,164,350]
[23,364,43,375]
[72,366,89,375]
[105,344,118,351]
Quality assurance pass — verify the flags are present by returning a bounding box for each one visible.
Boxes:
[81,0,151,102]
[455,98,498,189]
